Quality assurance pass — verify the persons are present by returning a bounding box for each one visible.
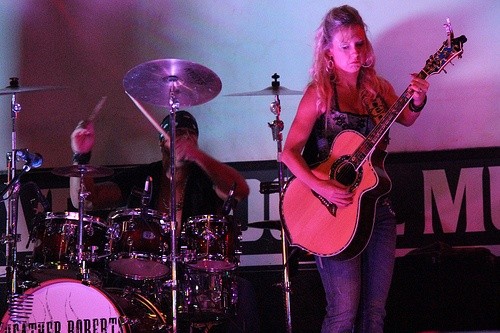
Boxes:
[279,4,430,333]
[69,110,262,333]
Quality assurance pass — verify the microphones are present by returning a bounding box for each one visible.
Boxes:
[223,183,237,211]
[141,176,153,207]
[16,149,43,168]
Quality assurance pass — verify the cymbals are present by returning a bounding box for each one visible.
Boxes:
[122,58,222,108]
[52,164,114,177]
[0,83,66,95]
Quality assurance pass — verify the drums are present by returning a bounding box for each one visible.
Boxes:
[0,277,167,333]
[184,214,238,265]
[179,255,237,314]
[108,207,173,280]
[37,211,108,266]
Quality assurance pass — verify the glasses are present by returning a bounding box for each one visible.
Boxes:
[174,128,197,137]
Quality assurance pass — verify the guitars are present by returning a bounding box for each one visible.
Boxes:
[278,18,467,263]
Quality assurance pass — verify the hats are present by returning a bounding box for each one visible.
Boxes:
[158,110,199,139]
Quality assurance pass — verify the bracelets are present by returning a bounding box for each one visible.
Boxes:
[410,95,427,112]
[71,151,92,164]
